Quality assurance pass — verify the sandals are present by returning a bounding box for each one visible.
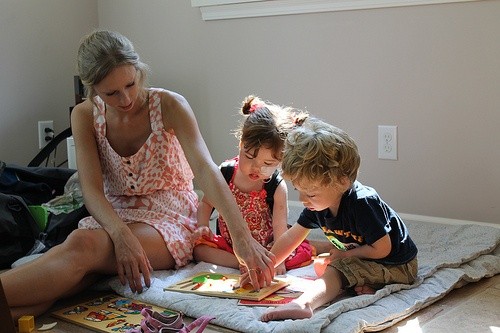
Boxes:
[124,307,184,333]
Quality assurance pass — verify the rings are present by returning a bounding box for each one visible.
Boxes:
[248,268,257,272]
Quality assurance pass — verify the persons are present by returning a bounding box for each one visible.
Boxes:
[0,31,277,325]
[240,117,418,321]
[193,98,315,275]
